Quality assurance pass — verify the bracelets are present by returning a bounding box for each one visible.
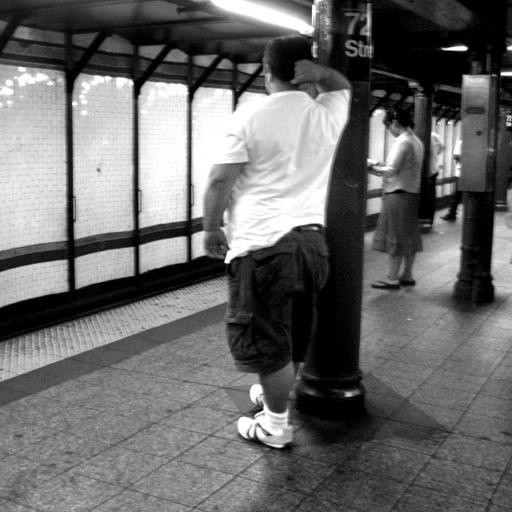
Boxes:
[368,163,374,171]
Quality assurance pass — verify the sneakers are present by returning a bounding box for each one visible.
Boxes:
[249,382,266,407]
[236,414,294,449]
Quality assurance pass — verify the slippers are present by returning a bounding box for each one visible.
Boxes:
[370,276,415,289]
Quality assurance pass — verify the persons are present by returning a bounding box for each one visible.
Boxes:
[426,130,448,226]
[439,137,463,223]
[366,106,426,291]
[201,34,354,451]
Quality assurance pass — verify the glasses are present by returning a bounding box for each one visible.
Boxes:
[385,123,392,128]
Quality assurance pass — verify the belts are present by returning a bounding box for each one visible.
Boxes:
[294,223,322,231]
